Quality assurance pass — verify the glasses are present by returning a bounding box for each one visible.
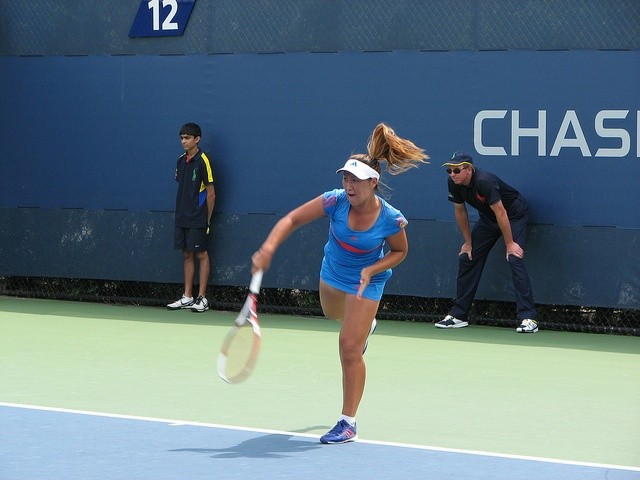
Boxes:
[447,166,468,173]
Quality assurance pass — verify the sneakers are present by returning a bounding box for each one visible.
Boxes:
[167,294,194,309]
[362,317,377,355]
[191,296,209,312]
[435,314,468,328]
[516,318,539,333]
[320,419,357,443]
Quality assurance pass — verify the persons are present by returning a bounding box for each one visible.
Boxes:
[167,122,216,312]
[435,152,540,333]
[251,122,430,444]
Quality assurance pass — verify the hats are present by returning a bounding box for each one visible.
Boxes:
[336,159,380,181]
[442,152,473,166]
